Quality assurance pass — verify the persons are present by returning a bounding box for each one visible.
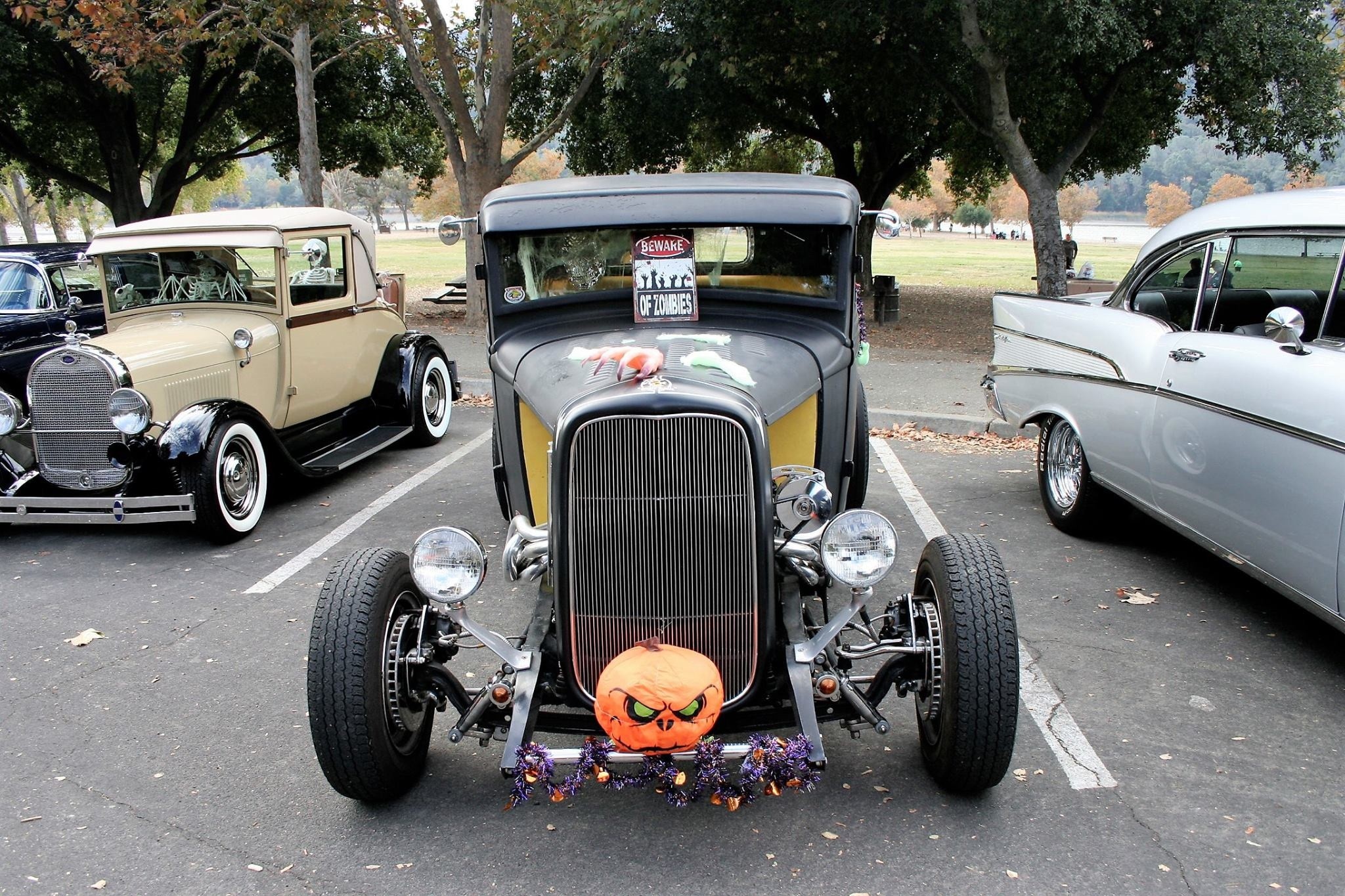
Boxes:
[1063,233,1078,270]
[1183,247,1243,289]
[901,223,1026,240]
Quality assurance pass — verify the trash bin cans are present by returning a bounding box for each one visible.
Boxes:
[380,225,391,234]
[872,275,900,326]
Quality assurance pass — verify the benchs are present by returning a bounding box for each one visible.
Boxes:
[1134,289,1318,342]
[248,283,343,305]
[423,274,466,304]
[544,274,828,296]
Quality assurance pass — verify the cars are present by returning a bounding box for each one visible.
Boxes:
[0,207,464,549]
[305,171,1019,803]
[980,186,1345,634]
